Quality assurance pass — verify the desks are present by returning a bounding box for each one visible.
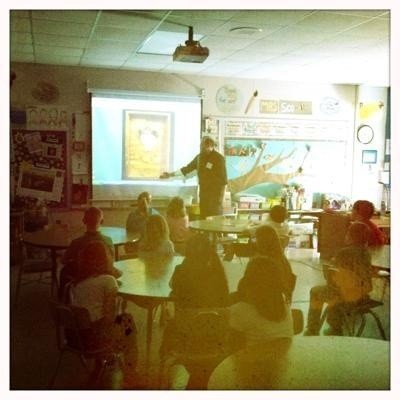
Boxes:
[87,196,170,230]
[369,244,390,269]
[233,207,324,230]
[369,215,391,246]
[187,218,259,264]
[112,255,248,365]
[207,335,391,390]
[23,223,141,298]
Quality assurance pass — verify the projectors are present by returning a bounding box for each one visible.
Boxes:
[172,26,209,64]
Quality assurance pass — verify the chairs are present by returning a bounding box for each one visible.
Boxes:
[50,304,126,390]
[367,268,389,304]
[315,263,387,340]
[14,232,59,301]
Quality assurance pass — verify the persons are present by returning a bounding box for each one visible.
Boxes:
[63,240,151,389]
[169,235,228,309]
[351,200,387,245]
[60,207,114,265]
[237,226,293,293]
[250,206,291,253]
[159,136,226,220]
[228,257,293,340]
[303,222,372,335]
[138,215,174,256]
[126,192,160,231]
[165,197,188,243]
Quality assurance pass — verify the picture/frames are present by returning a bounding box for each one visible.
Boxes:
[357,125,374,145]
[361,150,377,164]
